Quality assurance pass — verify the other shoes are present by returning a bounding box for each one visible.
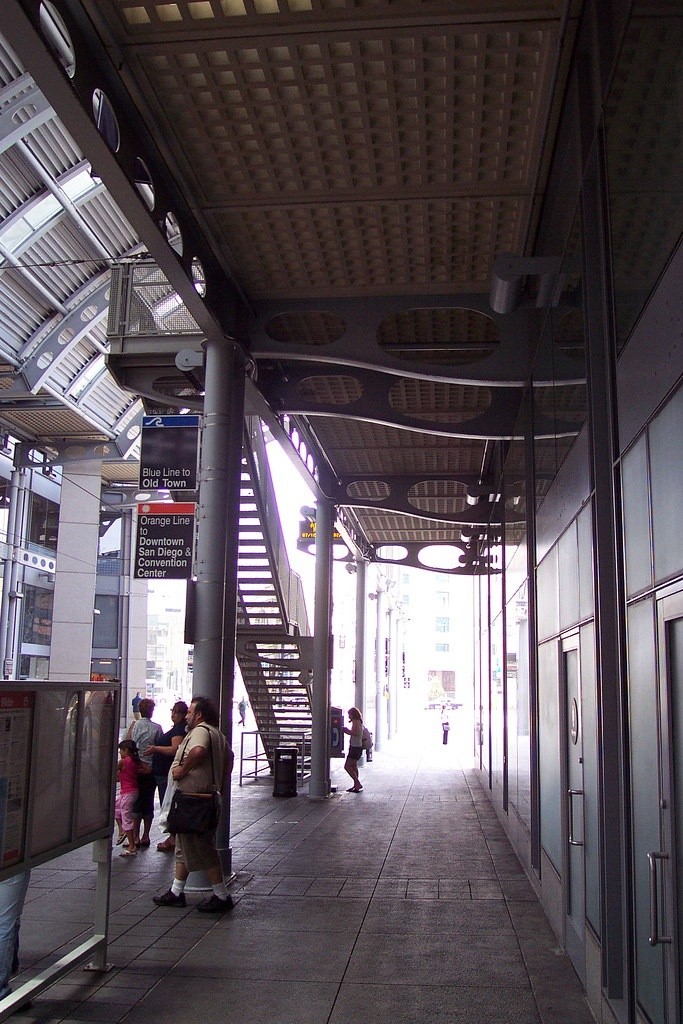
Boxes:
[157,838,176,851]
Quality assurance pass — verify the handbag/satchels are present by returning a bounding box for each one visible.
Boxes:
[162,789,221,837]
[116,757,121,782]
[362,724,373,749]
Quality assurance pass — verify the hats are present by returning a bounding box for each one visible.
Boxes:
[170,701,188,714]
[118,740,139,750]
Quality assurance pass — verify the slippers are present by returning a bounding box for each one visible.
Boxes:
[347,787,359,791]
[115,832,127,845]
[118,850,136,856]
[350,788,363,792]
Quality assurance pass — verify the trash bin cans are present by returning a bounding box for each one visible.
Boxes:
[273,748,298,797]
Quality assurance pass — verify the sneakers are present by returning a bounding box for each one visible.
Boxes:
[152,888,186,907]
[197,894,234,913]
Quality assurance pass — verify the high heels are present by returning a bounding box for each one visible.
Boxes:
[136,838,150,847]
[123,840,140,848]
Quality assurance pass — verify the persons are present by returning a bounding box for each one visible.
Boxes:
[343,708,363,792]
[153,696,234,913]
[237,697,246,726]
[114,692,188,856]
[82,706,100,758]
[440,705,449,744]
[0,870,32,1001]
